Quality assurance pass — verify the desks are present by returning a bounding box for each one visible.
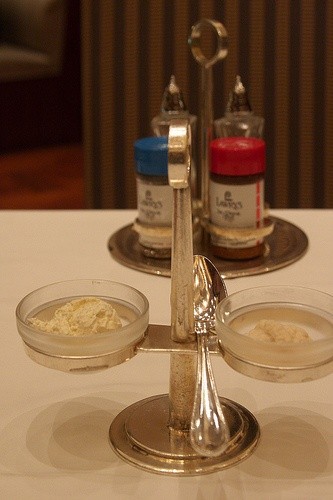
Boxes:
[0,209,333,500]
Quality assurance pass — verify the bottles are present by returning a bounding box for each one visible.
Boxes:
[150,75,197,137]
[212,76,264,140]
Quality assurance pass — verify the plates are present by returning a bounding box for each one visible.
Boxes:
[108,208,310,280]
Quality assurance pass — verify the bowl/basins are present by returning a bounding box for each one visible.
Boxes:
[214,285,333,383]
[17,277,150,372]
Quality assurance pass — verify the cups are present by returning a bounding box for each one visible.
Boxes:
[207,136,267,261]
[132,138,190,258]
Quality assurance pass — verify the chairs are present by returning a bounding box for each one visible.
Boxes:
[0,0,80,157]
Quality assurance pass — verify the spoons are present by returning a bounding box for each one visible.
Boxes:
[190,255,233,456]
